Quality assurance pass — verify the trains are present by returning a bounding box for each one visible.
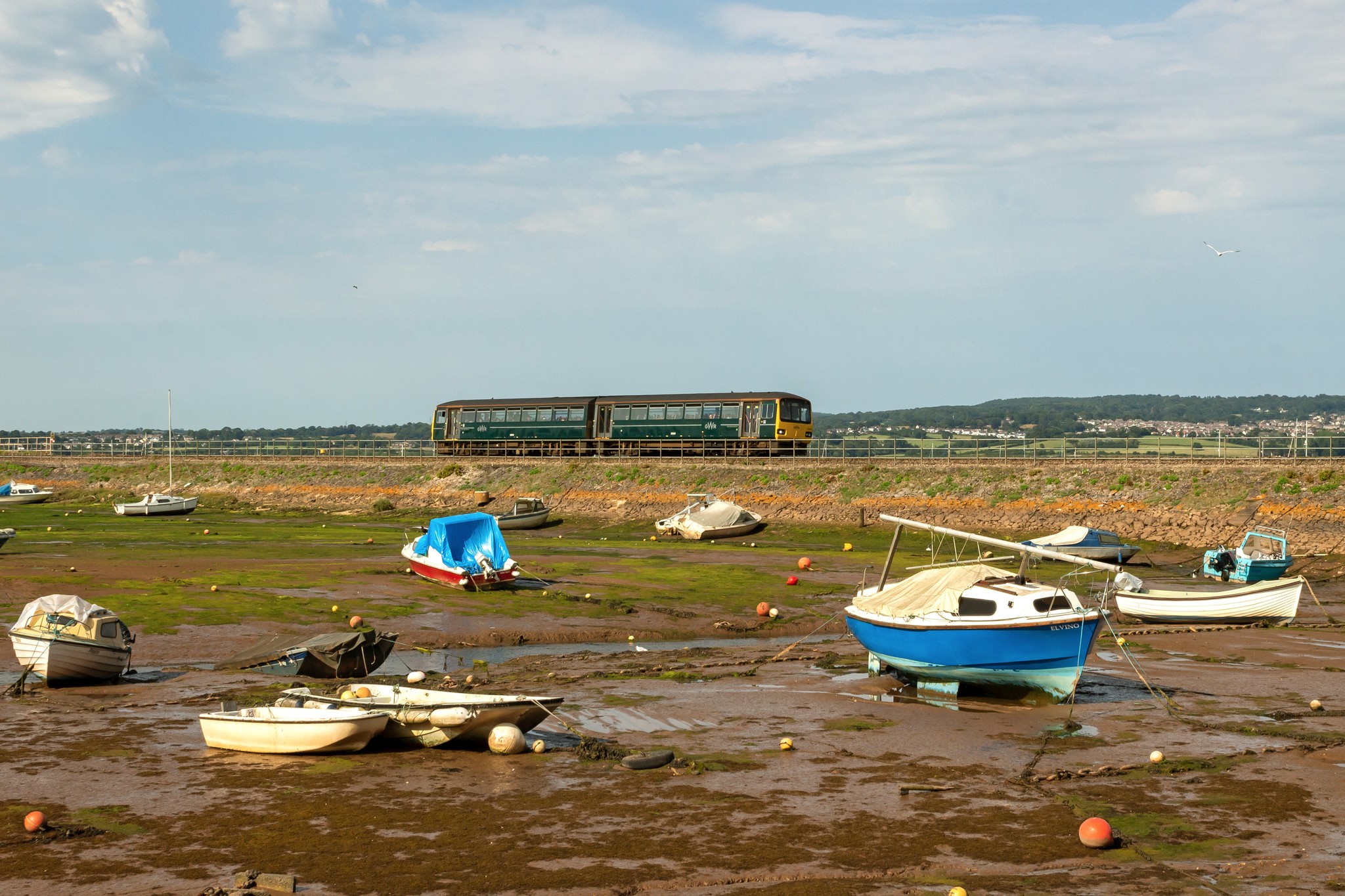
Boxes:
[431,391,814,457]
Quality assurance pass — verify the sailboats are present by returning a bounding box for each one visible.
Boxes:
[111,388,207,517]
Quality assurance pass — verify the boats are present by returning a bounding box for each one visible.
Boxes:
[401,511,519,592]
[8,594,129,683]
[655,488,763,541]
[844,512,1128,707]
[1203,512,1295,585]
[493,494,554,531]
[0,478,54,505]
[1095,571,1308,629]
[280,683,566,755]
[198,701,393,754]
[213,627,402,680]
[0,528,19,549]
[1015,525,1142,566]
